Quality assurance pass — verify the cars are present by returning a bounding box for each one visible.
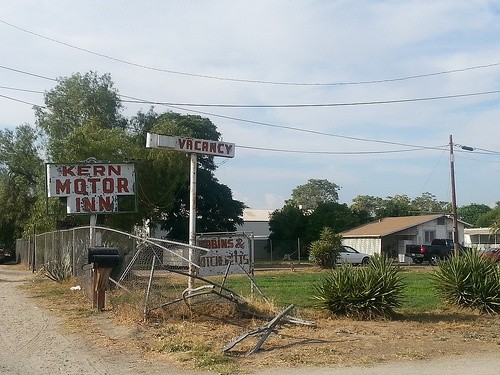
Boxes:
[309,244,374,266]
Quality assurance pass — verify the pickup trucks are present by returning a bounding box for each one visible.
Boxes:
[406,235,474,265]
